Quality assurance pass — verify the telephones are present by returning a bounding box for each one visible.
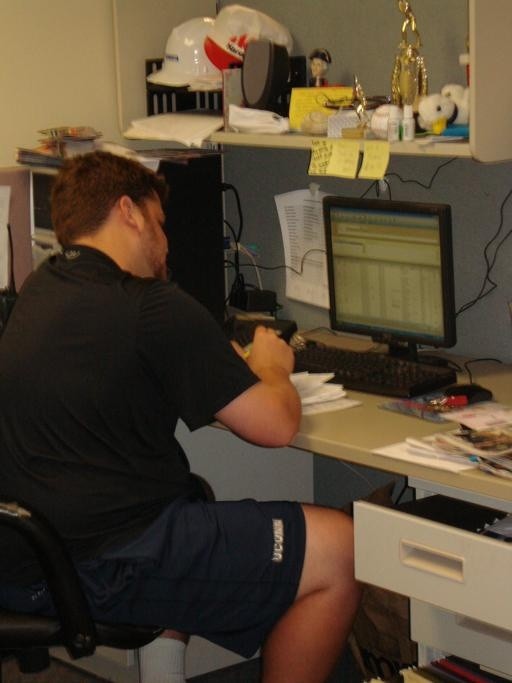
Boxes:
[167,270,176,286]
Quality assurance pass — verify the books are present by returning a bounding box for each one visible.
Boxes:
[119,110,224,148]
[290,367,510,483]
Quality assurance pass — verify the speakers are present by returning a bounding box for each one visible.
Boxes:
[241,40,290,117]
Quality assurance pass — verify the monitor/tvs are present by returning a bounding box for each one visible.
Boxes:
[322,196,462,373]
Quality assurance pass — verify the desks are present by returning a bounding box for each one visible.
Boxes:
[207,326,511,504]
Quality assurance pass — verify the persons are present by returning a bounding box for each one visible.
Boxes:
[0,151,362,683]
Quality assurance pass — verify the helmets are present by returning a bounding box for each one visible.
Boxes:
[146,16,222,87]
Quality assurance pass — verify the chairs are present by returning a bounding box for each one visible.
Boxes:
[1,497,167,682]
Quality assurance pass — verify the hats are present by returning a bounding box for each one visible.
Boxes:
[203,4,293,70]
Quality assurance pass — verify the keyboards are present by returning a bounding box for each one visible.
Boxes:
[293,344,457,398]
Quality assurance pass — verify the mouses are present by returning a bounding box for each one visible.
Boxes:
[447,383,492,403]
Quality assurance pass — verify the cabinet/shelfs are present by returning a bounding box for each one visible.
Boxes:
[351,472,511,683]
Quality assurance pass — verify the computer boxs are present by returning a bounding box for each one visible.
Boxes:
[29,154,225,328]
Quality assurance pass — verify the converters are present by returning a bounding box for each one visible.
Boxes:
[230,289,278,311]
